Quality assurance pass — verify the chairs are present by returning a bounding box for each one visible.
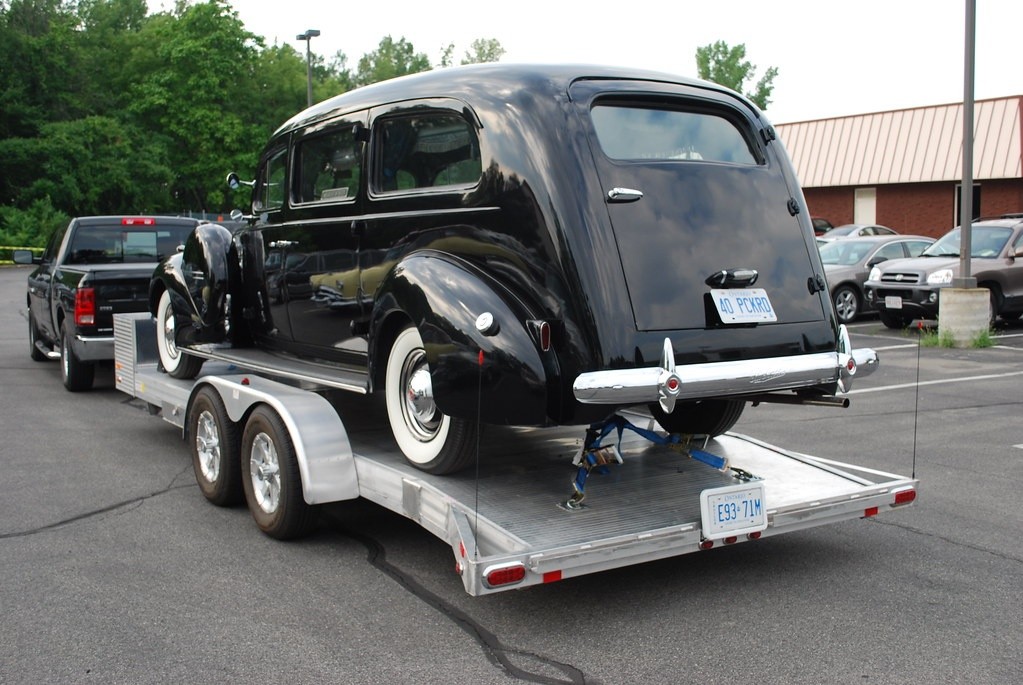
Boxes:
[75,237,106,263]
[156,236,181,263]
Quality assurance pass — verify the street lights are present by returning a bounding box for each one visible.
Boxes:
[296,28,322,106]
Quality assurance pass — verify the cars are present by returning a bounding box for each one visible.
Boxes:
[812,222,902,237]
[810,216,834,236]
[13,216,218,393]
[819,234,959,325]
[143,63,880,476]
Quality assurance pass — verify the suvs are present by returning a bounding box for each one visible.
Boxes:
[861,212,1023,333]
